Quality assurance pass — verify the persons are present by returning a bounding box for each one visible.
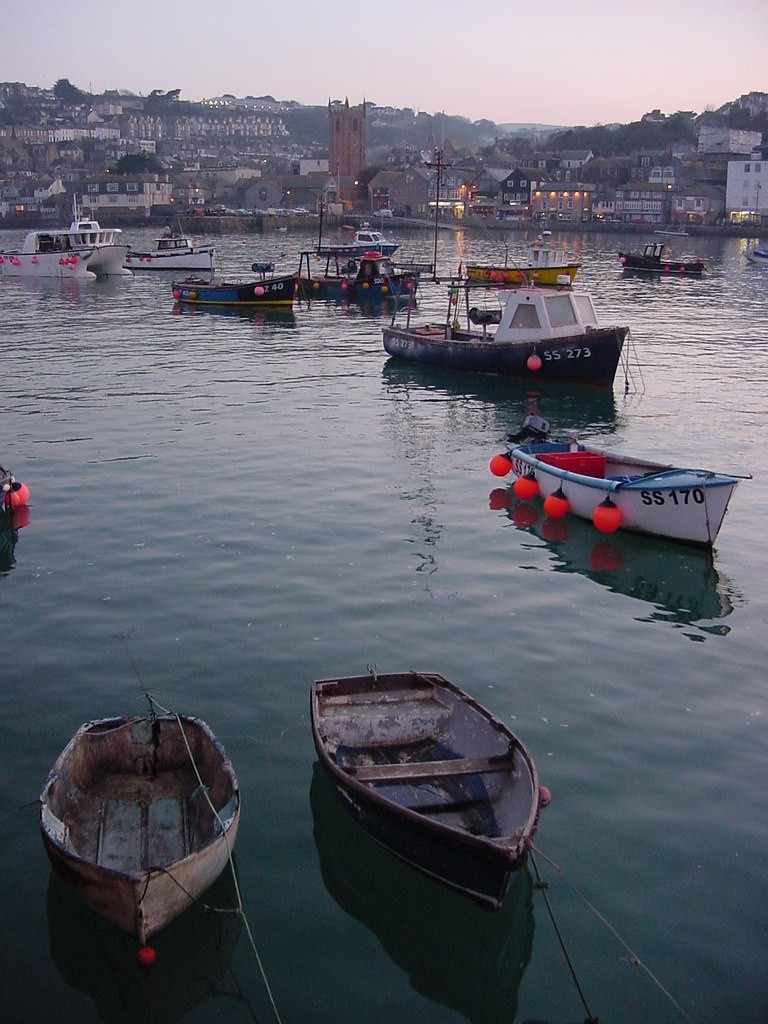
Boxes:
[195,206,201,216]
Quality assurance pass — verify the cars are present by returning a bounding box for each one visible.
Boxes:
[373,210,392,218]
[187,203,309,217]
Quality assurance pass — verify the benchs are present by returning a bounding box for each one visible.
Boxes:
[347,753,516,782]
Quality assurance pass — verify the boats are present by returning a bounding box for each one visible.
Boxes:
[354,257,434,272]
[508,416,740,549]
[309,671,546,907]
[122,225,216,270]
[0,192,132,279]
[466,238,582,288]
[744,249,768,264]
[170,270,296,306]
[37,714,241,943]
[618,243,703,275]
[380,283,631,386]
[314,229,401,257]
[278,200,419,300]
[655,228,688,236]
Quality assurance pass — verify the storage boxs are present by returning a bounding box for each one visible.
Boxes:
[535,451,607,479]
